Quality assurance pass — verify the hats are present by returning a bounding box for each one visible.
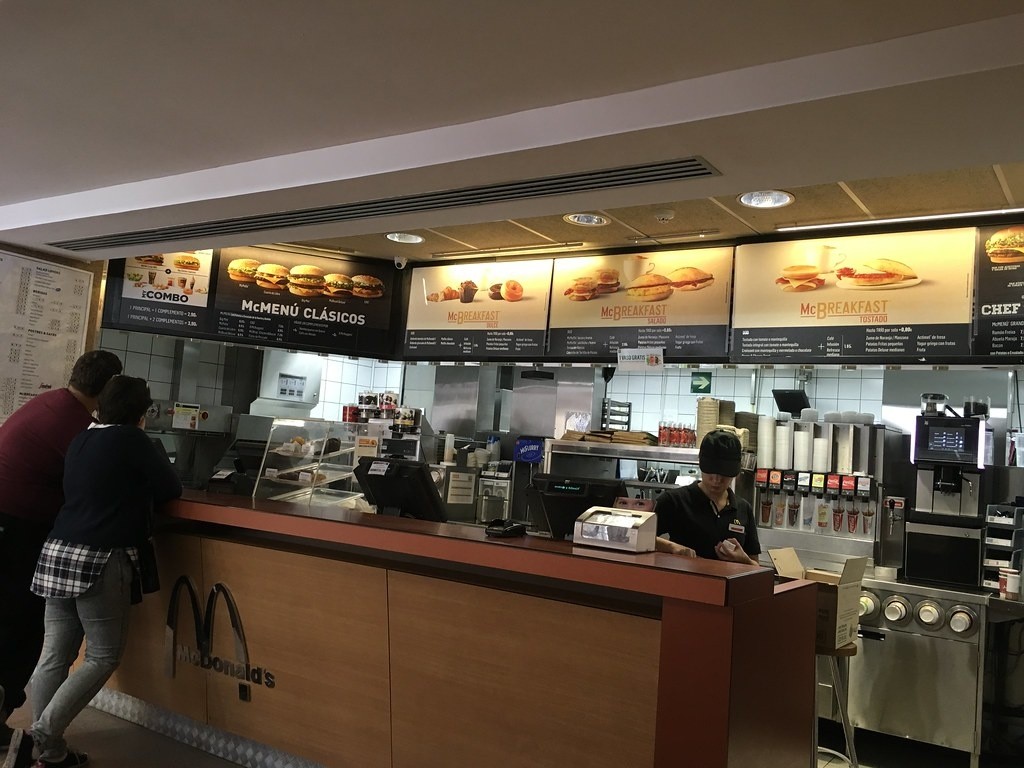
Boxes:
[699,429,742,478]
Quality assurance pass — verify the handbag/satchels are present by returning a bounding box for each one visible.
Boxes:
[136,535,161,594]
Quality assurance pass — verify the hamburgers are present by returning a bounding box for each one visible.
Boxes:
[174,256,200,271]
[322,274,354,298]
[564,277,598,301]
[593,269,620,293]
[351,275,384,298]
[775,266,825,292]
[226,259,261,283]
[287,264,325,296]
[134,253,164,266]
[254,264,290,290]
[669,267,714,290]
[840,257,918,286]
[985,225,1024,263]
[624,273,673,302]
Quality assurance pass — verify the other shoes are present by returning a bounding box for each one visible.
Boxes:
[0,722,14,749]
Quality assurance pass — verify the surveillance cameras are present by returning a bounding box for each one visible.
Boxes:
[394,257,407,269]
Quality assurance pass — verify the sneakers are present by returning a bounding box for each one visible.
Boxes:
[2,728,38,768]
[36,751,88,768]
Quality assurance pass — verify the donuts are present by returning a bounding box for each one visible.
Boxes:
[488,280,523,302]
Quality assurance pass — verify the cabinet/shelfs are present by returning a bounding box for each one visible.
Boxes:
[252,417,366,509]
[980,504,1023,594]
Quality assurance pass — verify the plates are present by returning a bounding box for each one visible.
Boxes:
[837,277,922,290]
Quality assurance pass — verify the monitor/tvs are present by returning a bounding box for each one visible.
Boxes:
[525,473,630,540]
[352,455,449,524]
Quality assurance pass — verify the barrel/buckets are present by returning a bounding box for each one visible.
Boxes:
[342,390,415,425]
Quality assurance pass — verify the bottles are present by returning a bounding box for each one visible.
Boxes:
[658,421,696,446]
[1008,429,1018,466]
[984,429,994,466]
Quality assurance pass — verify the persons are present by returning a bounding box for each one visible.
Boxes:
[0,349,124,749]
[614,497,654,512]
[1,374,185,767]
[655,431,762,566]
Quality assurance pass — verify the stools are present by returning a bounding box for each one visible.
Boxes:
[813,643,862,768]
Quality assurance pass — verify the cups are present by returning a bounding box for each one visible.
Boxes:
[443,433,455,463]
[847,512,860,533]
[807,246,846,274]
[788,503,798,527]
[855,597,972,635]
[803,504,813,527]
[761,500,773,522]
[775,503,785,525]
[486,435,500,462]
[623,258,656,282]
[1014,432,1024,467]
[863,513,874,534]
[818,504,829,527]
[833,509,844,532]
[999,567,1021,598]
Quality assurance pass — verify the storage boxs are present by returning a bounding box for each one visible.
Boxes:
[767,546,869,653]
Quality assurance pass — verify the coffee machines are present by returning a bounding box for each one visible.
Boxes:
[911,414,988,526]
